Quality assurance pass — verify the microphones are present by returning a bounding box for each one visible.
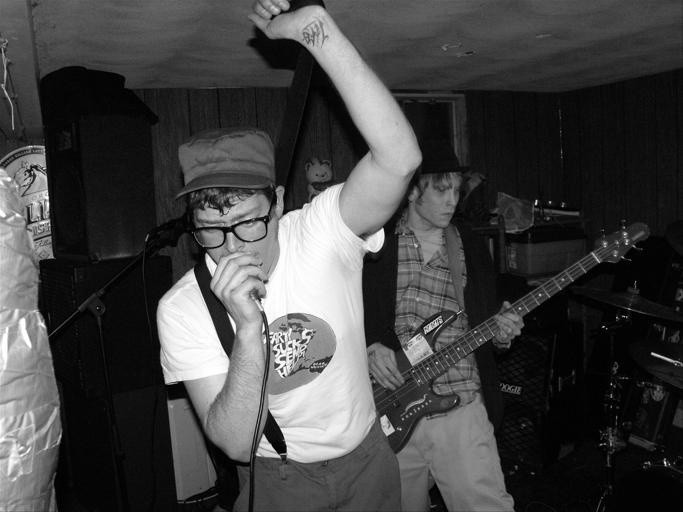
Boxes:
[249,289,266,314]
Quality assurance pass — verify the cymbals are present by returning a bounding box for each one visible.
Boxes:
[630,342,681,390]
[565,285,679,320]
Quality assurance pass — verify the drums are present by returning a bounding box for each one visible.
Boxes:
[628,380,683,453]
[594,461,683,512]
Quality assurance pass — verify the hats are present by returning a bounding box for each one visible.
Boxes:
[415,138,465,175]
[173,129,276,201]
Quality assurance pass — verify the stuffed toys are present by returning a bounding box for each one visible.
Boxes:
[305,160,333,202]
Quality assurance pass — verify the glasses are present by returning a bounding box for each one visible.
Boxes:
[188,192,277,249]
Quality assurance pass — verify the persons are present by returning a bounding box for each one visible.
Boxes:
[362,144,525,512]
[155,0,425,512]
[599,218,683,359]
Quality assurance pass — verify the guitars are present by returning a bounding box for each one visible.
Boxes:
[371,221,655,452]
